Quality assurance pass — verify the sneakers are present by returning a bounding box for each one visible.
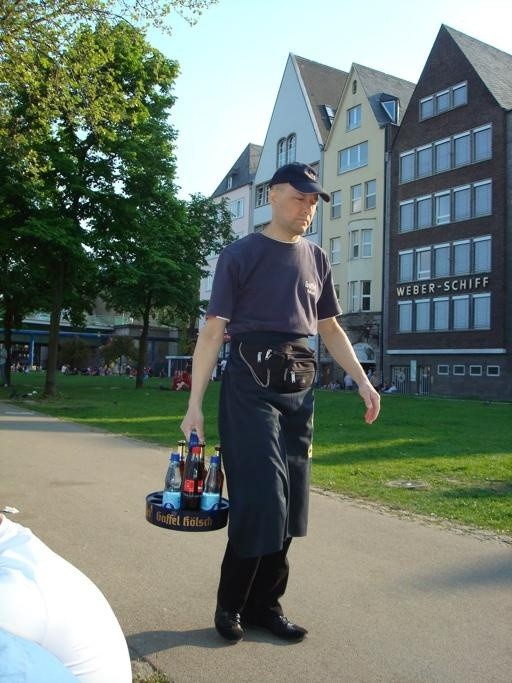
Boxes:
[214,603,243,644]
[239,614,306,642]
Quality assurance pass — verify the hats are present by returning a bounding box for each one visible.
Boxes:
[268,161,331,202]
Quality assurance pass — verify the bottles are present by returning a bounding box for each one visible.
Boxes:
[161,440,224,512]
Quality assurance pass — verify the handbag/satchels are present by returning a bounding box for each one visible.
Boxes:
[232,338,318,393]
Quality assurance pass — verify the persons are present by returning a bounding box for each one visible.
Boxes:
[61,364,112,376]
[160,368,165,377]
[334,371,397,393]
[125,364,153,377]
[181,163,381,641]
[218,358,227,375]
[0,341,8,387]
